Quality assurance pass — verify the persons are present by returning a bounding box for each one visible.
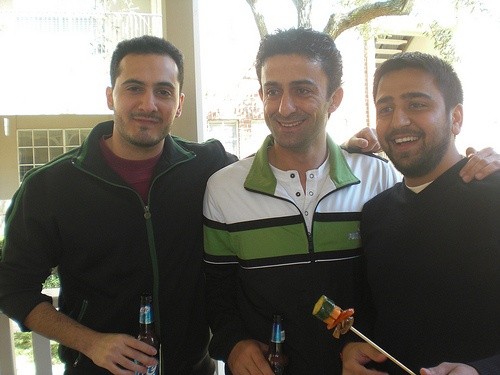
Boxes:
[202,26,500,375]
[341,51,500,375]
[0,35,383,375]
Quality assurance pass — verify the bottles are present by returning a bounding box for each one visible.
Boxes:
[135,294,159,375]
[266,312,290,375]
